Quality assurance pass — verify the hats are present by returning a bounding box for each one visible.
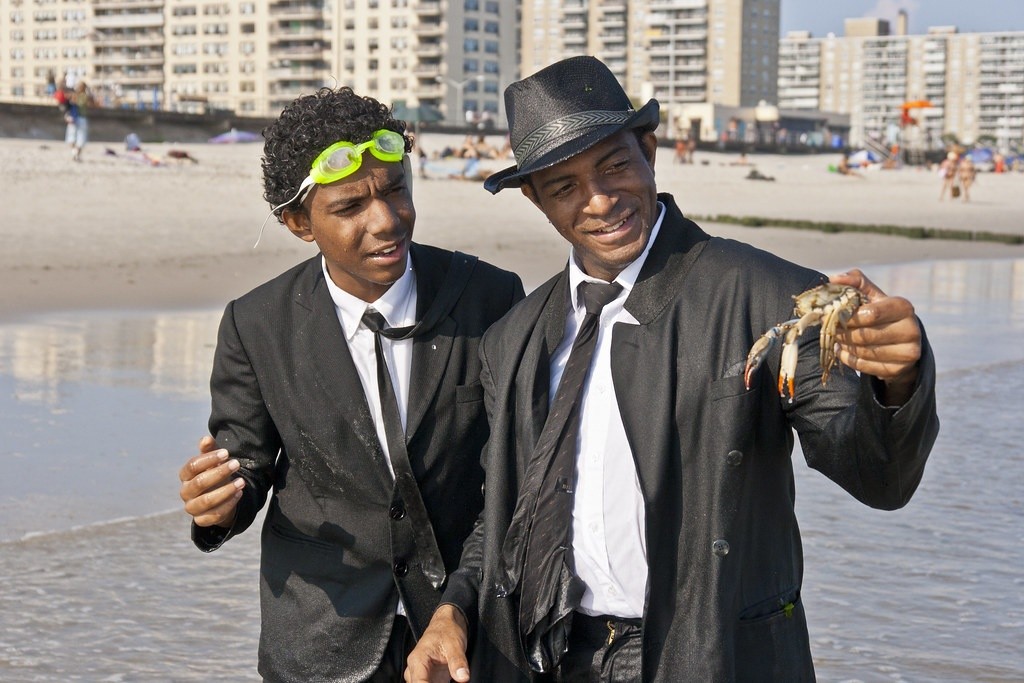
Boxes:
[483,58,662,197]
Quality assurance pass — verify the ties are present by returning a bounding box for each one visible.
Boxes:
[499,281,626,636]
[360,252,446,589]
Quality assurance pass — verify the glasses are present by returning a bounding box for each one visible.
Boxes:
[295,128,406,208]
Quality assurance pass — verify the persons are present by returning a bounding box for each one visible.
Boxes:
[178,72,529,683]
[402,54,941,683]
[43,70,1023,204]
